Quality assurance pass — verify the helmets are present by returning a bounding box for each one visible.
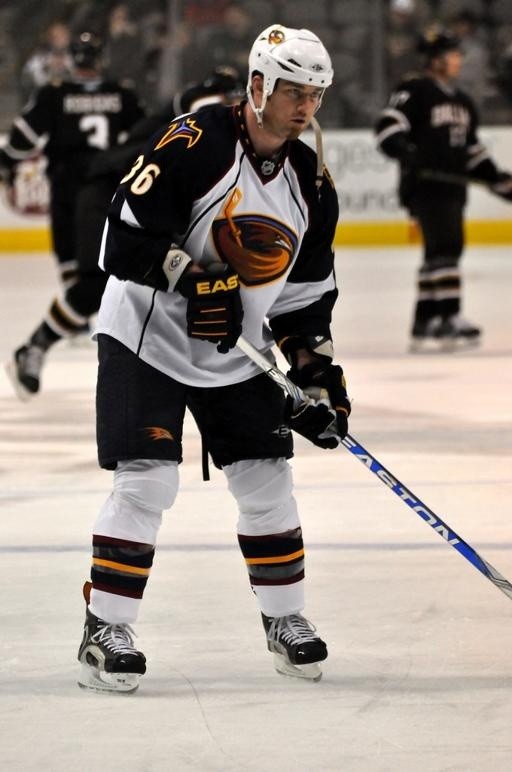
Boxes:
[66,12,114,72]
[419,27,461,62]
[246,23,334,130]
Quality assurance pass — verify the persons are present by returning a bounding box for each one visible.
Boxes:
[1,1,511,212]
[72,22,354,676]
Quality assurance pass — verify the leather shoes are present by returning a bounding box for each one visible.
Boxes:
[260,614,329,666]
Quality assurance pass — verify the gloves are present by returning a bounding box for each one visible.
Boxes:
[182,259,245,355]
[283,363,353,451]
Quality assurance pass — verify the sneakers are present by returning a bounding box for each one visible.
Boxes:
[12,342,45,395]
[412,317,482,340]
[77,581,147,677]
